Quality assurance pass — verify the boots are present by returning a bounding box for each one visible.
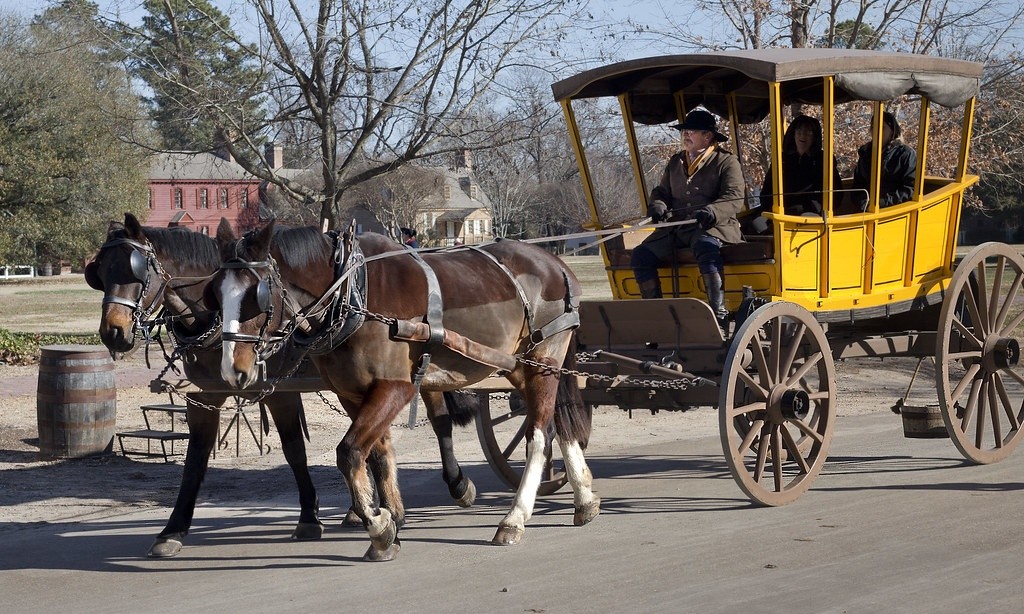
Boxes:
[637,272,663,299]
[701,273,730,328]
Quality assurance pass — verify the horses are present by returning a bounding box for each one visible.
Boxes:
[91,212,479,562]
[214,215,601,562]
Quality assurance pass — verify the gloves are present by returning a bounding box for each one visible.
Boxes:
[685,207,716,230]
[646,198,671,222]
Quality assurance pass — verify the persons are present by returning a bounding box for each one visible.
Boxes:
[400,227,420,248]
[852,110,918,210]
[752,114,840,257]
[454,237,463,246]
[631,112,746,325]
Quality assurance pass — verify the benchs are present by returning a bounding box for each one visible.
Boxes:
[606,232,775,267]
[837,174,956,217]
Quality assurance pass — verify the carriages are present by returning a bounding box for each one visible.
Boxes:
[83,48,1024,560]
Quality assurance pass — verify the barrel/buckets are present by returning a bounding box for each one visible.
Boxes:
[37,344,118,458]
[891,356,965,439]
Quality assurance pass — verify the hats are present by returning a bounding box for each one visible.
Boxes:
[668,111,728,143]
[400,227,416,237]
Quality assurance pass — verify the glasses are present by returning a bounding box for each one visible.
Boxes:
[401,231,408,235]
[678,128,696,137]
